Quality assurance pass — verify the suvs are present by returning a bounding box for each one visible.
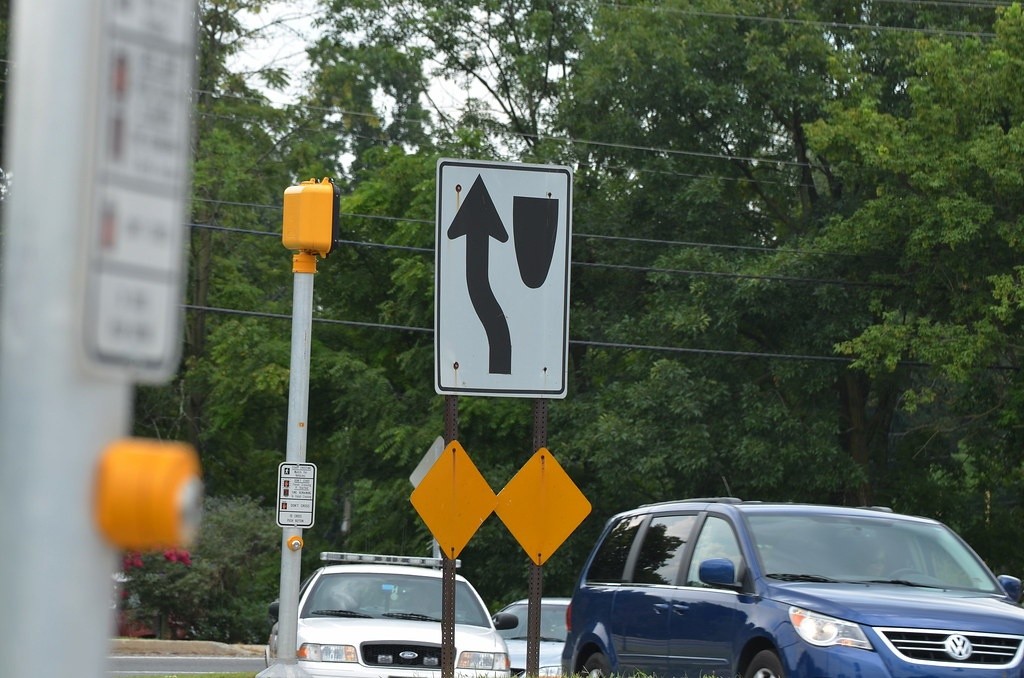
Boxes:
[561,497,1024,678]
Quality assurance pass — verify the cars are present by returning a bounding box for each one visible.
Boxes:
[263,552,519,678]
[492,596,572,678]
